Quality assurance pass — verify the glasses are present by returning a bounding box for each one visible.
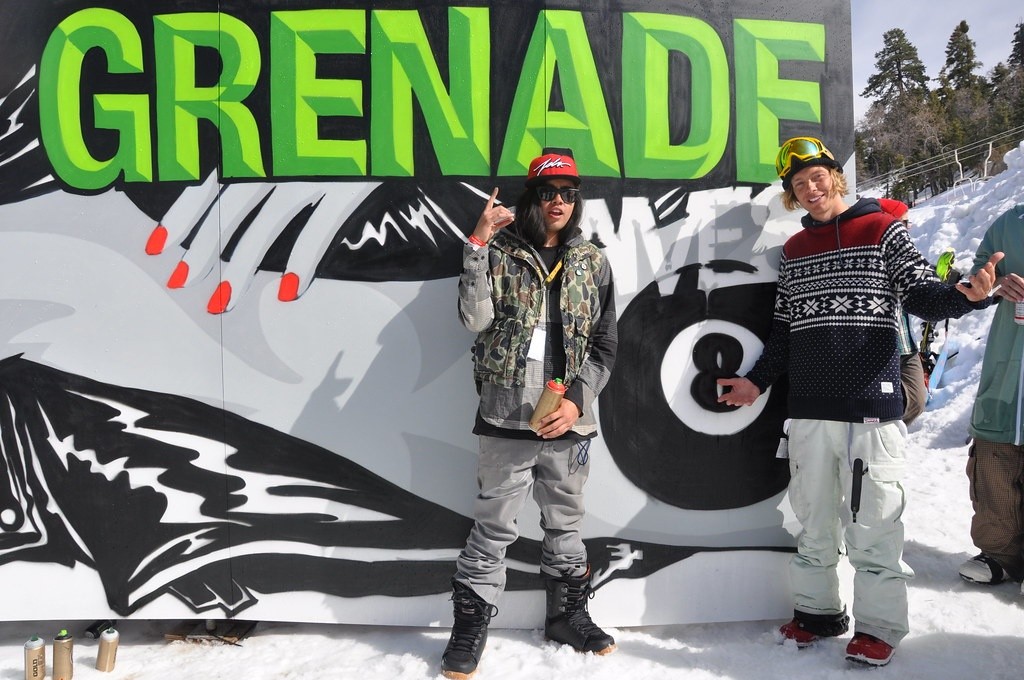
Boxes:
[535,184,579,204]
[776,137,835,177]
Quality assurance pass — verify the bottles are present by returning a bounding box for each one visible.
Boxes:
[528,378,565,435]
[53,629,73,680]
[95,627,120,672]
[24,634,45,680]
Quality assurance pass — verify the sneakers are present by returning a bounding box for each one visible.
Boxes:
[778,619,821,648]
[959,552,1010,585]
[846,633,895,667]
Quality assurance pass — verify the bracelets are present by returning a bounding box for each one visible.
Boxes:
[469,235,485,246]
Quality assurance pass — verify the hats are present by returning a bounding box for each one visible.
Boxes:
[776,138,843,190]
[524,154,582,188]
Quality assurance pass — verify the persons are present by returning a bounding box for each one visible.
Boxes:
[440,153,618,680]
[717,137,1004,667]
[878,199,927,426]
[957,201,1024,592]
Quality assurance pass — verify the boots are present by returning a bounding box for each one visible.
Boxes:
[441,577,498,680]
[540,563,616,656]
